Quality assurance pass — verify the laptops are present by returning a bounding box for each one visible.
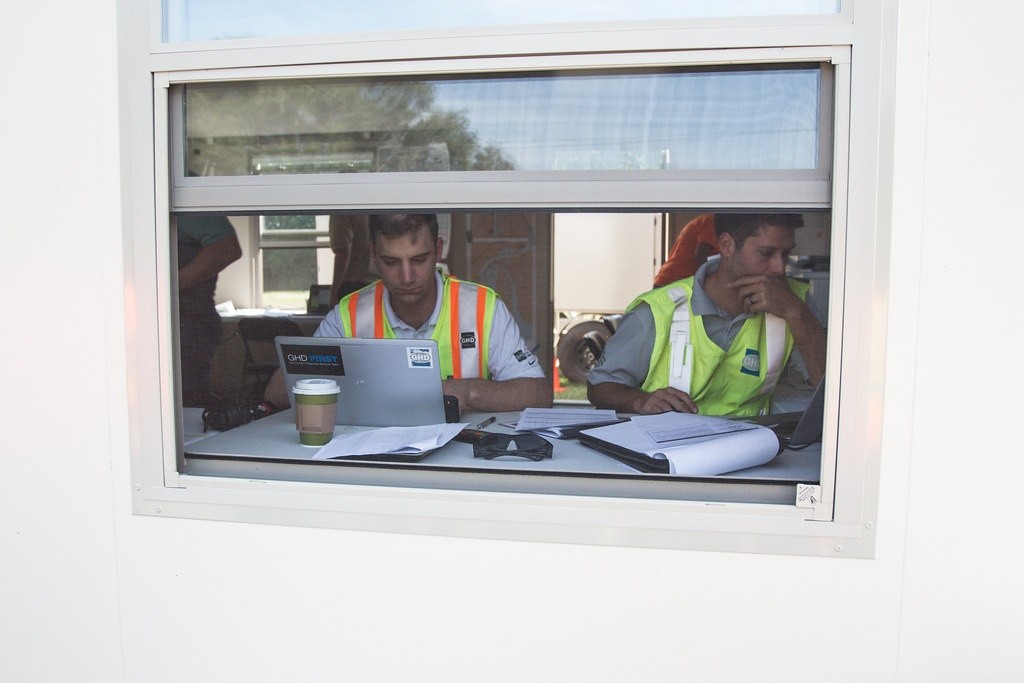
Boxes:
[293,284,331,318]
[274,336,447,427]
[728,374,826,450]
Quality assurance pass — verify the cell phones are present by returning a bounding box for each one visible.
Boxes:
[442,395,460,423]
[453,429,490,444]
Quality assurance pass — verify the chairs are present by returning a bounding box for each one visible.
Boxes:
[238,318,303,401]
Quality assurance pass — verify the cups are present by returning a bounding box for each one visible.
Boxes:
[292,379,341,448]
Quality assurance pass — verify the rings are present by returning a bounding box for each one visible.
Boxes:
[748,295,754,305]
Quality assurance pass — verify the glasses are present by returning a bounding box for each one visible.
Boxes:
[473,432,553,461]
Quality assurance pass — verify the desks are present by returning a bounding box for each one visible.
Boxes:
[184,309,824,506]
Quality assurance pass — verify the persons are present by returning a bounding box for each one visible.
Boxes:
[264,213,552,415]
[586,213,827,414]
[177,171,242,408]
[653,213,720,289]
[329,214,381,306]
